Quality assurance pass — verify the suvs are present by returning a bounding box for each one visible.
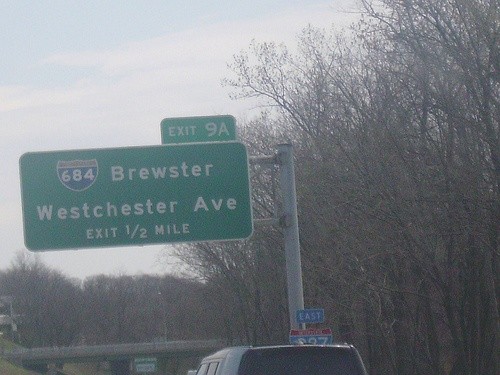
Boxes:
[188,342,370,375]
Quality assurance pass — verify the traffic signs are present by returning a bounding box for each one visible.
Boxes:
[19,114,253,252]
